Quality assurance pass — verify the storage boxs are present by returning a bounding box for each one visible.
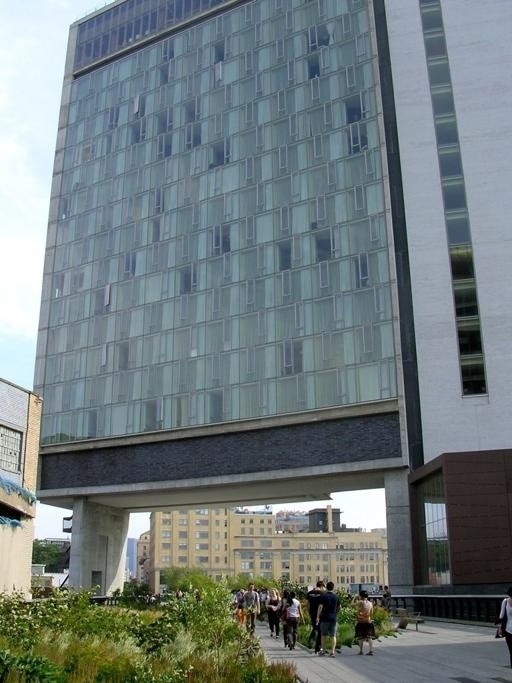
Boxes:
[396,607,425,631]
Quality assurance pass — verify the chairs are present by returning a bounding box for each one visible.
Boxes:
[283,642,296,651]
[270,633,279,639]
[314,649,335,657]
[358,651,374,655]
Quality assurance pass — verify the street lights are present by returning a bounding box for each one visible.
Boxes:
[357,612,372,623]
[494,628,507,638]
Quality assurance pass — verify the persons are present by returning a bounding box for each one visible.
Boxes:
[497,586,511,668]
[230,578,392,658]
